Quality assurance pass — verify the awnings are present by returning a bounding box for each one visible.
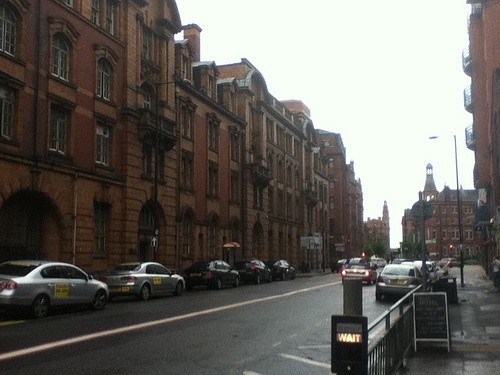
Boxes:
[223,242,240,248]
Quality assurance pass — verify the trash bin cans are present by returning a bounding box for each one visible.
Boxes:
[434,276,458,305]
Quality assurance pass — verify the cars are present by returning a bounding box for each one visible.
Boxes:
[440,258,461,267]
[234,258,273,285]
[185,260,241,291]
[374,265,425,298]
[372,257,389,268]
[342,258,378,286]
[101,262,186,301]
[0,262,111,318]
[390,258,438,276]
[260,258,297,282]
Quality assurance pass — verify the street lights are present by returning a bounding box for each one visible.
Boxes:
[428,134,465,271]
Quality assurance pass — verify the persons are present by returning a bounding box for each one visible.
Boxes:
[492,256,500,291]
[372,263,378,283]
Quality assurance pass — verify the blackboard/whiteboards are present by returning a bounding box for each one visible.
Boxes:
[412,292,450,353]
